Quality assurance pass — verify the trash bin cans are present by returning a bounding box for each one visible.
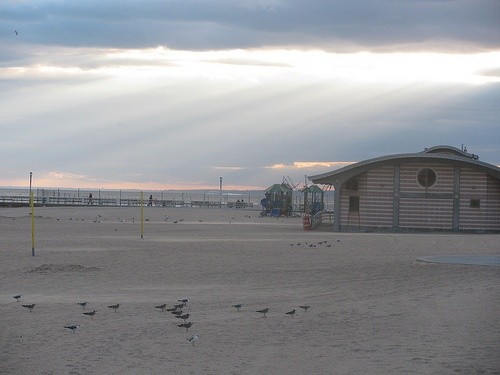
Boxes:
[272,208,281,218]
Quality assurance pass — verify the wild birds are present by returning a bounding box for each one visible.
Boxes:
[21,303,36,312]
[255,307,271,318]
[76,301,89,310]
[107,303,121,313]
[82,309,98,320]
[231,303,245,310]
[285,309,298,318]
[63,323,81,334]
[185,334,200,348]
[298,305,311,312]
[336,239,340,242]
[56,213,252,224]
[287,240,332,248]
[463,146,467,153]
[154,296,195,333]
[12,293,24,302]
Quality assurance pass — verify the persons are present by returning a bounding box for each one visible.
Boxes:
[87,192,94,204]
[236,200,244,209]
[147,195,153,206]
